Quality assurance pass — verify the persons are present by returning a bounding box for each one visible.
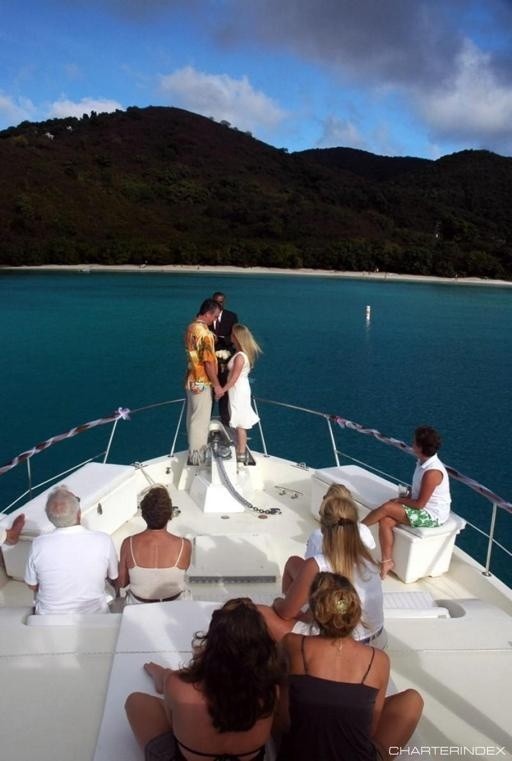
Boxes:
[183,298,225,467]
[206,291,238,446]
[23,488,119,619]
[0,513,25,546]
[215,324,264,467]
[116,485,193,608]
[123,597,294,761]
[269,570,424,761]
[254,482,388,650]
[358,427,451,581]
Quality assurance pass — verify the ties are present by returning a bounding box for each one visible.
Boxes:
[216,319,219,331]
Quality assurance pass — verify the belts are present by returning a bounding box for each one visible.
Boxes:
[129,589,184,603]
[358,628,384,644]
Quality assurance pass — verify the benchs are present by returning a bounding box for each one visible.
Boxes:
[0,459,149,586]
[308,464,467,586]
